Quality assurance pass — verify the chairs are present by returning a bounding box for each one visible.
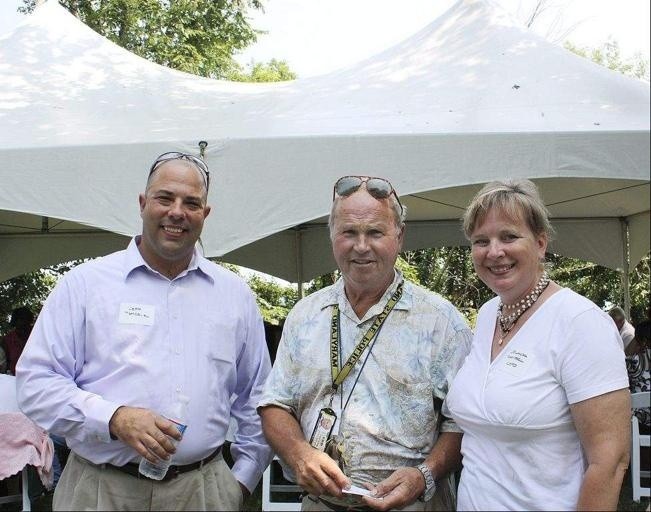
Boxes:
[628,392,651,503]
[0,372,33,510]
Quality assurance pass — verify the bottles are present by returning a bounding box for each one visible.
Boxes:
[140,394,192,480]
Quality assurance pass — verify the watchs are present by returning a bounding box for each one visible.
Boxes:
[414,464,436,503]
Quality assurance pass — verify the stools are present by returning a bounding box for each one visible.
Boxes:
[261,454,304,511]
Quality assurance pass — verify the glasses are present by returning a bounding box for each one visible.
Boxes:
[148,151,211,189]
[333,176,404,213]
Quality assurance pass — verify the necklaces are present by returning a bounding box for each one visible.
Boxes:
[496,271,550,345]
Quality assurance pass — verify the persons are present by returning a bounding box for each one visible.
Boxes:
[255,174,475,512]
[13,154,278,512]
[447,178,633,511]
[608,306,638,356]
[624,320,651,501]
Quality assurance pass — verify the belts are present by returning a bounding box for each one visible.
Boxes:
[117,445,221,483]
